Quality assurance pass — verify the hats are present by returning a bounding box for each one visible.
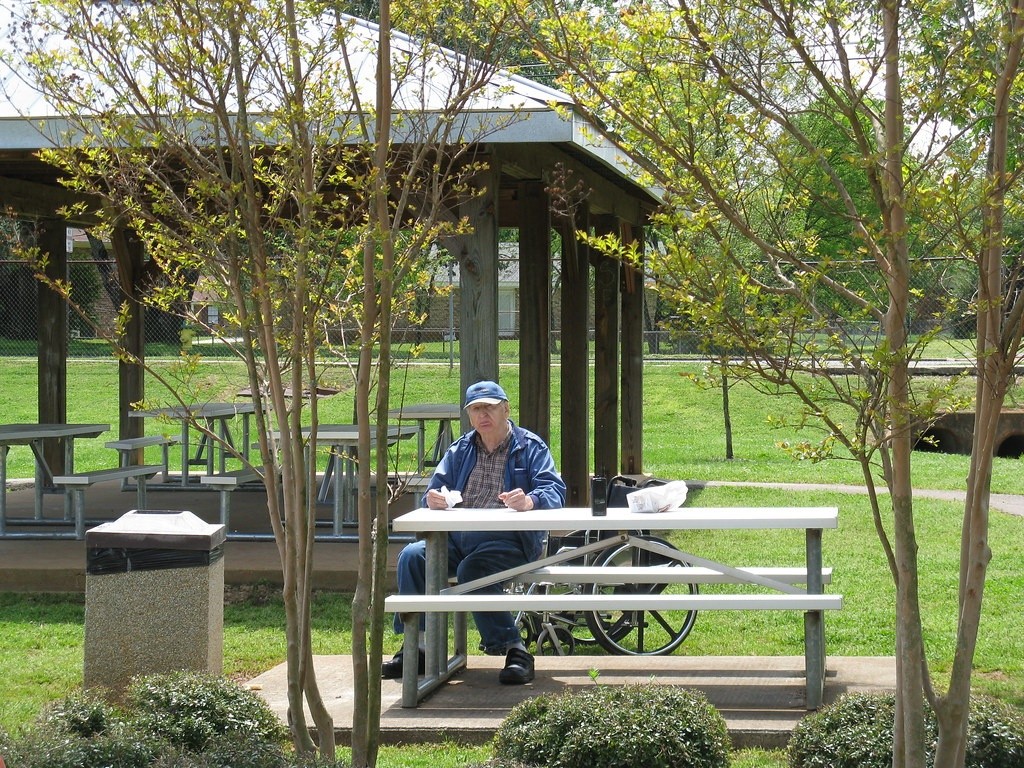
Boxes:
[463,381,508,409]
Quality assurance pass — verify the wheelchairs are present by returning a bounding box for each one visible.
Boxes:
[479,476,701,657]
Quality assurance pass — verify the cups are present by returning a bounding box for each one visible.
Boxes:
[591,477,606,516]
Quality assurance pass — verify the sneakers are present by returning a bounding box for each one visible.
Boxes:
[498,648,534,683]
[382,643,425,676]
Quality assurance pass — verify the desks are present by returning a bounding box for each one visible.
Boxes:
[0,423,119,540]
[127,403,266,491]
[393,506,839,687]
[230,423,419,541]
[372,403,461,485]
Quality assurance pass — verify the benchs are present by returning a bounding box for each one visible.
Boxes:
[250,442,284,490]
[104,435,182,491]
[397,470,434,550]
[53,465,166,541]
[384,595,844,712]
[447,567,833,673]
[201,465,283,541]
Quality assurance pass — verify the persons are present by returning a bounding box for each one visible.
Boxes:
[382,380,567,685]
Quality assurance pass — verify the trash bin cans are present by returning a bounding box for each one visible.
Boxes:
[82,507,226,699]
